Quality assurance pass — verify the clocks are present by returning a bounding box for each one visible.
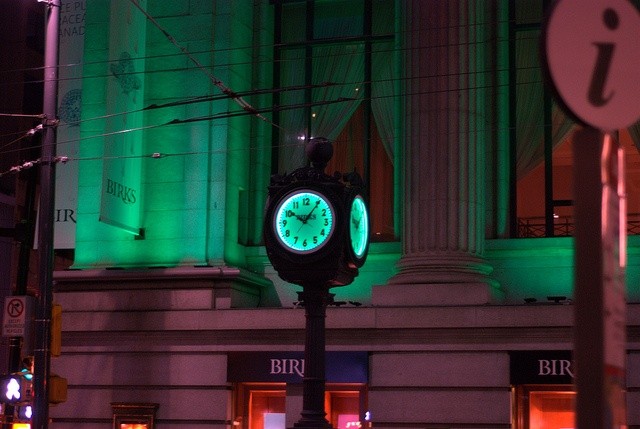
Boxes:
[273,189,336,254]
[349,195,370,258]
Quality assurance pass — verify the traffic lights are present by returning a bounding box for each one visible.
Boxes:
[16,402,33,420]
[1,374,27,404]
